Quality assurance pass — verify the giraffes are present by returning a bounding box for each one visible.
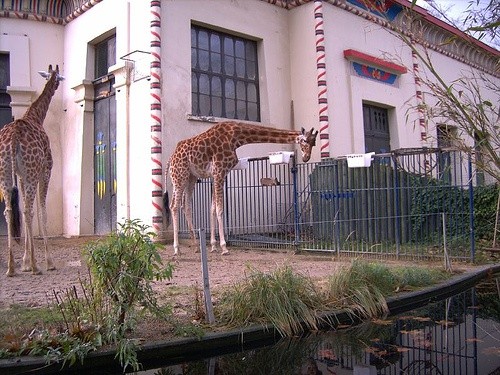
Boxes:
[0,64,66,277]
[162,121,318,256]
